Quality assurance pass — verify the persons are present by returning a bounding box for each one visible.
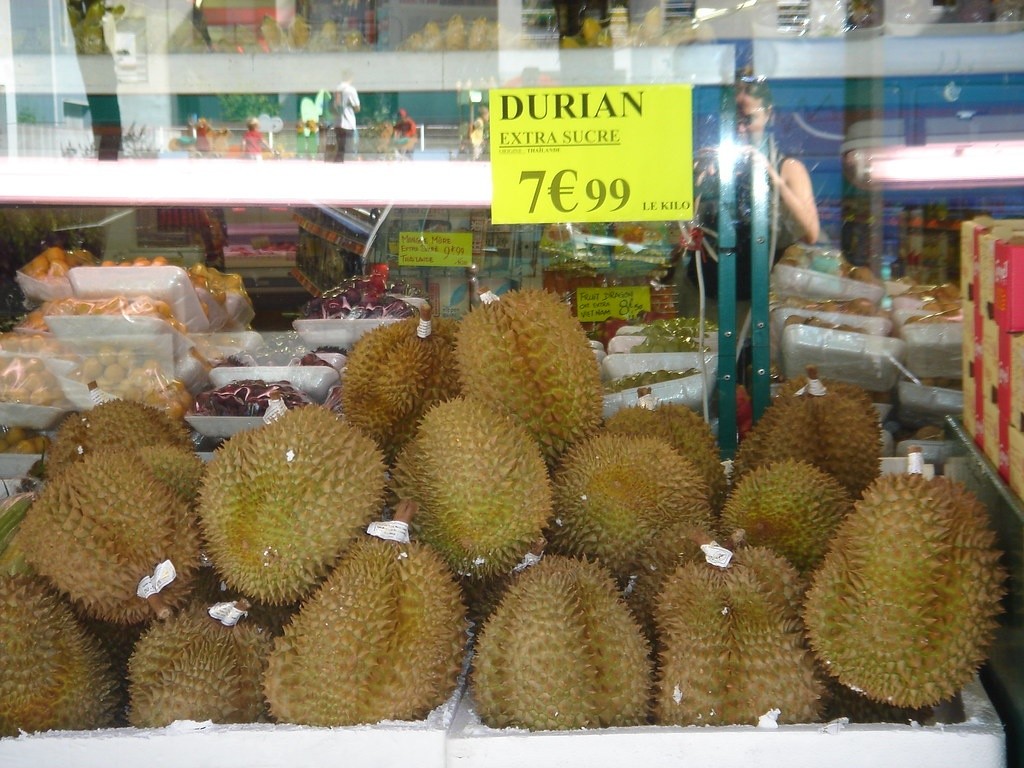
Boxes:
[467,105,490,158]
[693,68,821,297]
[329,70,360,161]
[393,108,417,160]
[243,118,270,160]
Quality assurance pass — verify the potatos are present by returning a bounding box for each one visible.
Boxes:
[0,247,254,458]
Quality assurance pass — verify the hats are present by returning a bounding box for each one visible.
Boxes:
[246,116,259,125]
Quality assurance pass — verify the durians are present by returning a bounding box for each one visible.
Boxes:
[0,288,1009,744]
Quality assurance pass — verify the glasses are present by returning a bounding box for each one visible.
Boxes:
[736,113,758,126]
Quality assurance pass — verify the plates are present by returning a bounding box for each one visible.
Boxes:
[0,240,966,484]
